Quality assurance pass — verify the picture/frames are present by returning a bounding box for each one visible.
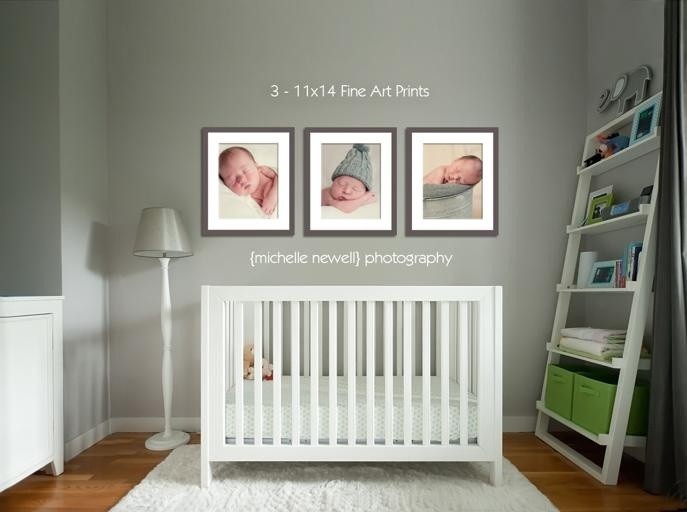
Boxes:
[200,127,295,237]
[587,259,617,288]
[303,127,397,237]
[628,94,661,147]
[405,127,498,237]
[585,184,614,224]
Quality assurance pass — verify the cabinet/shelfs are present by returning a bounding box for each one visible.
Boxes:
[1,313,55,494]
[534,90,663,486]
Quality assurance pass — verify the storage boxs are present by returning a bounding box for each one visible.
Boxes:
[545,362,650,436]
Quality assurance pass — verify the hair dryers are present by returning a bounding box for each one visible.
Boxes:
[576,251,598,287]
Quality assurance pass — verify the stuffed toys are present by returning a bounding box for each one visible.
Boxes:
[240,343,253,380]
[594,131,629,160]
[245,356,273,381]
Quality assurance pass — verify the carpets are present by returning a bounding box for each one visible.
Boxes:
[106,445,559,512]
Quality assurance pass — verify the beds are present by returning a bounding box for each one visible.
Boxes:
[199,285,504,487]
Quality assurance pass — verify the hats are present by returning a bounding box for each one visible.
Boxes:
[331,144,373,190]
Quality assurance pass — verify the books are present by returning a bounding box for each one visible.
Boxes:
[613,240,642,287]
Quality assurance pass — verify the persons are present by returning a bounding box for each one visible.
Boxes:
[320,144,378,215]
[217,146,278,215]
[422,154,481,186]
[593,206,601,217]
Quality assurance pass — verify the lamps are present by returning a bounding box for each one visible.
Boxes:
[132,206,195,452]
